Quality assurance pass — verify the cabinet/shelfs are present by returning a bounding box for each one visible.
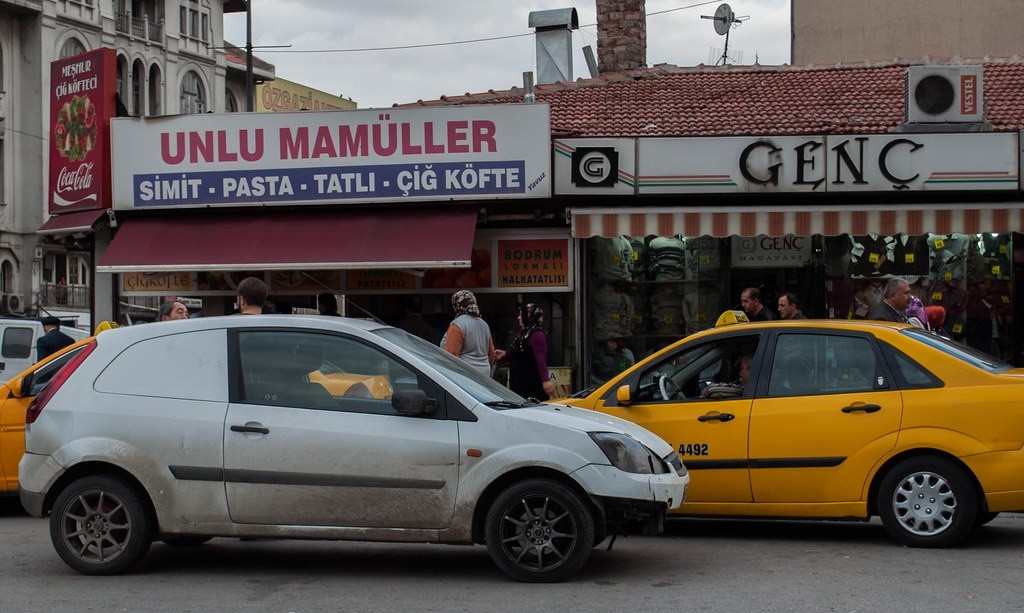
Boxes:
[589,235,726,388]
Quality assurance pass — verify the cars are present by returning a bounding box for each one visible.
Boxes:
[16,313,690,582]
[542,310,1024,549]
[0,319,394,492]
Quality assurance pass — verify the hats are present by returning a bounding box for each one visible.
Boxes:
[41,316,63,325]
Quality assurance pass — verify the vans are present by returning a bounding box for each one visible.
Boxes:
[0,321,91,388]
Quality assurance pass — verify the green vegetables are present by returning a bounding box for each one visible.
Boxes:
[56,98,97,161]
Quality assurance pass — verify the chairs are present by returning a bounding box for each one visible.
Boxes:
[789,357,816,395]
[241,336,342,411]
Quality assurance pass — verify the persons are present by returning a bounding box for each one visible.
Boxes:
[495,304,555,405]
[439,290,495,378]
[244,333,284,387]
[59,276,65,303]
[230,276,267,315]
[740,288,771,321]
[778,294,806,319]
[868,278,912,325]
[848,279,885,320]
[700,355,753,398]
[37,316,75,363]
[157,302,188,321]
[317,292,342,317]
[904,294,930,331]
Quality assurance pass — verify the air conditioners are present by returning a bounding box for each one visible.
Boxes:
[3,294,25,312]
[904,65,983,124]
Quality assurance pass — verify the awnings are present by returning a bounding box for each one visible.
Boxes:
[95,208,478,274]
[35,209,106,234]
